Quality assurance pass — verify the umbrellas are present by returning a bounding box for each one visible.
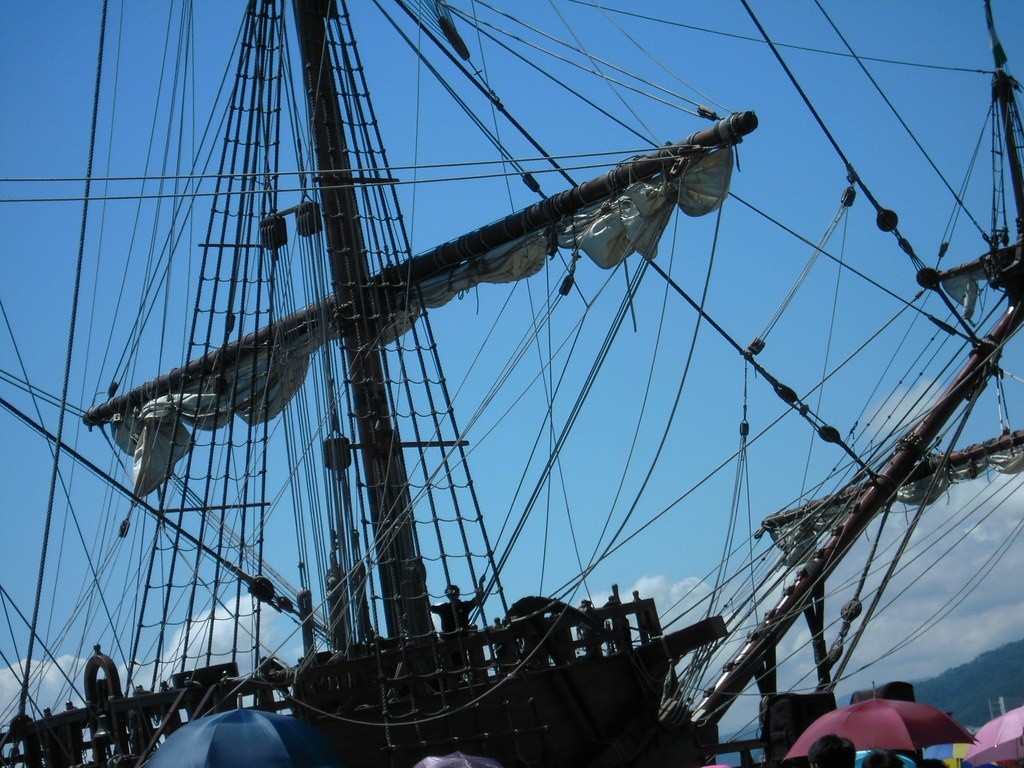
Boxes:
[781,682,982,760]
[412,750,504,768]
[143,693,353,768]
[856,705,1024,768]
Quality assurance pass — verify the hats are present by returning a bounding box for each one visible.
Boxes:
[445,586,459,597]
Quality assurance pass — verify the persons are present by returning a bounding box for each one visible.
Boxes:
[807,733,950,768]
[430,577,484,691]
